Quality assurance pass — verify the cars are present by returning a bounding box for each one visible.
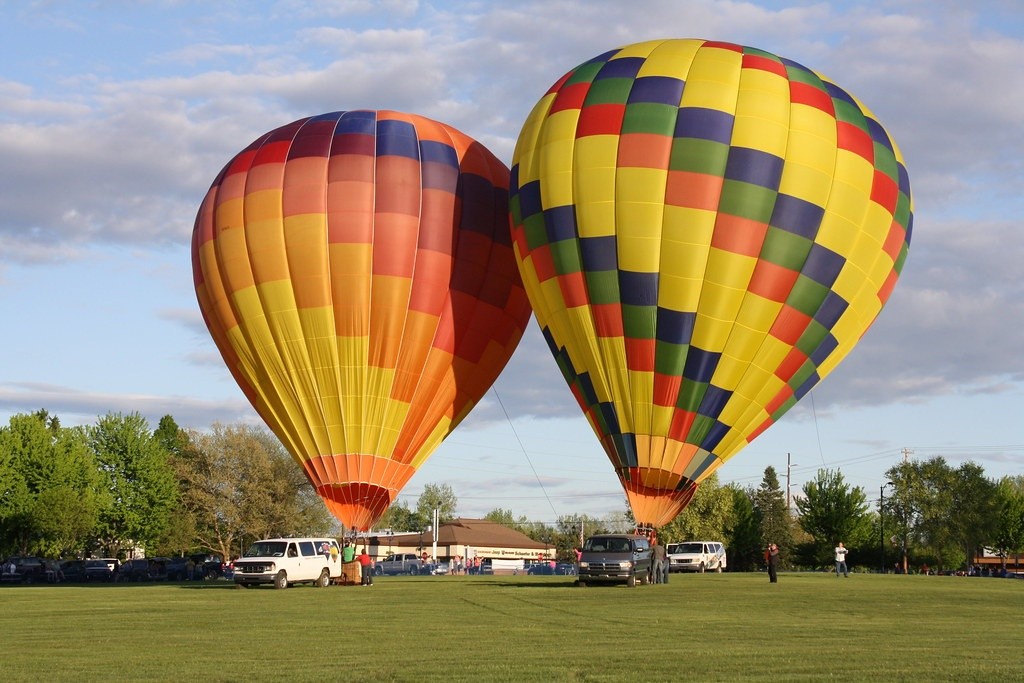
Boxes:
[6,553,222,585]
[527,559,577,575]
[469,561,492,575]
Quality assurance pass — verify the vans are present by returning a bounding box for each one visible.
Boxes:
[578,533,652,588]
[233,537,342,588]
[666,541,728,574]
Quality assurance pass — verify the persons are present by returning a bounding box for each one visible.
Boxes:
[835,542,849,578]
[341,538,484,587]
[651,541,670,584]
[0,559,237,584]
[895,562,1007,578]
[513,548,582,575]
[767,544,779,583]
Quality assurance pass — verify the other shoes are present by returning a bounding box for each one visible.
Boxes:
[836,575,839,578]
[186,579,189,581]
[363,584,367,586]
[845,576,849,578]
[371,584,373,586]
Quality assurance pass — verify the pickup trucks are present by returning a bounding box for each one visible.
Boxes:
[375,553,449,576]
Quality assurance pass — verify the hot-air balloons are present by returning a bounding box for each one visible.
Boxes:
[190,110,531,584]
[507,37,915,550]
